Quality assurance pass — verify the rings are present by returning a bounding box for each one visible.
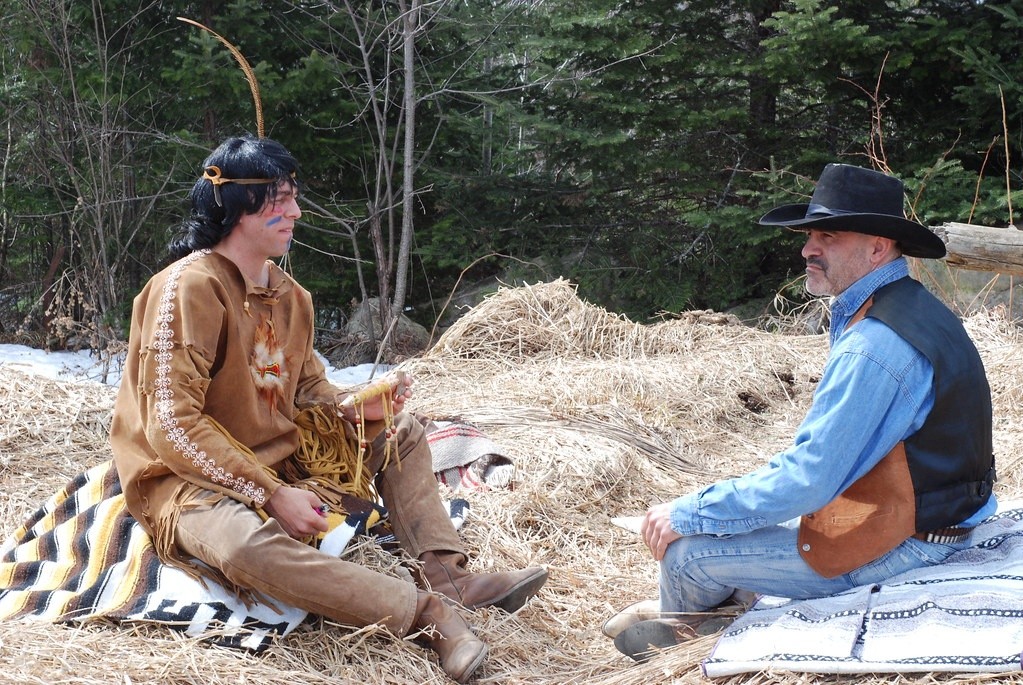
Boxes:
[395,394,400,401]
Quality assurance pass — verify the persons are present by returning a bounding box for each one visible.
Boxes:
[110,137,548,684]
[602,163,997,665]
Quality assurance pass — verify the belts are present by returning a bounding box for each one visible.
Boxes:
[911,527,972,543]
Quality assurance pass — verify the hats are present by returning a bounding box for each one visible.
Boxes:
[758,162,947,258]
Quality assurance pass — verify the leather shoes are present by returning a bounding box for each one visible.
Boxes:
[602,587,756,640]
[614,608,747,663]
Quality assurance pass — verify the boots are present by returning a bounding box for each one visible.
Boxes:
[405,587,488,684]
[413,550,548,614]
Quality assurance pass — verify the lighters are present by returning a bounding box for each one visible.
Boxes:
[315,504,329,514]
